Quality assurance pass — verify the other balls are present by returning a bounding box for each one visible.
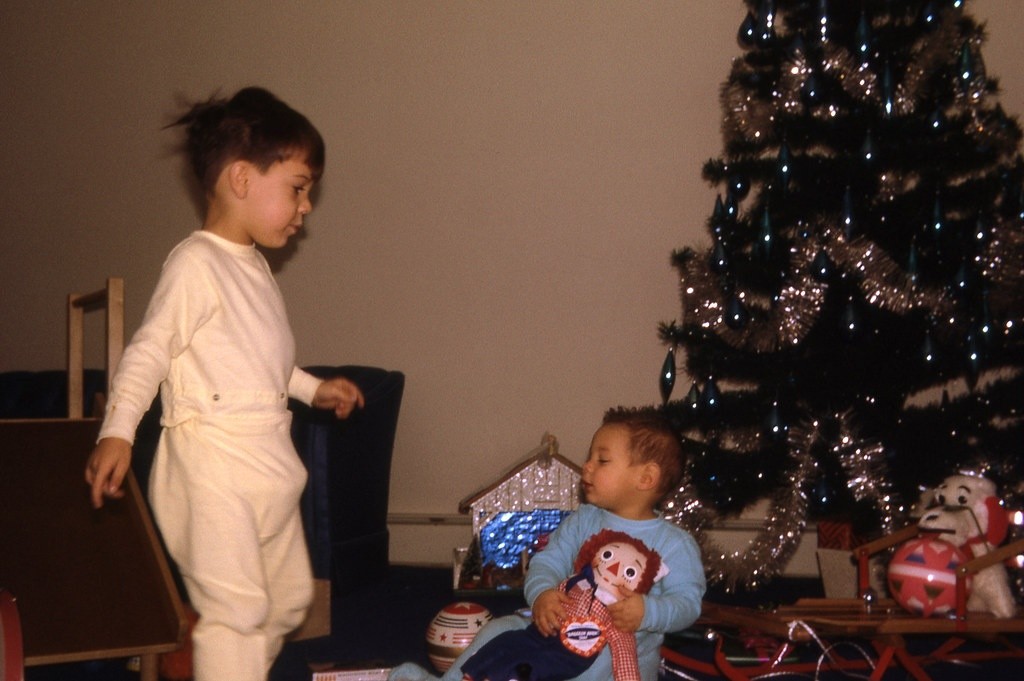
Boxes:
[425,602,497,676]
[886,536,973,618]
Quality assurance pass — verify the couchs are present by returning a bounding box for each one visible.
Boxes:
[0,364,405,680]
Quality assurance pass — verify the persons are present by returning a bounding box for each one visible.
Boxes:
[392,406,705,681]
[88,82,362,681]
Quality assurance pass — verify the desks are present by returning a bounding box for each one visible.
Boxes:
[658,601,1023,681]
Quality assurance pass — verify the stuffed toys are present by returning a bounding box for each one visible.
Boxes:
[460,529,670,680]
[917,475,1017,620]
